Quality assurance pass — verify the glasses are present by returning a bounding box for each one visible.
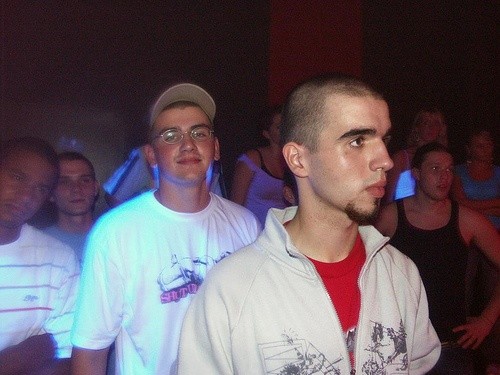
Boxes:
[151,126,214,148]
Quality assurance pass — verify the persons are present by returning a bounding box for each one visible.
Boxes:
[71,81,259,375]
[176,73,442,375]
[36,105,500,268]
[0,137,81,375]
[371,142,500,375]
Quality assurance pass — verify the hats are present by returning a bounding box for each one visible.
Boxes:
[143,84,216,139]
